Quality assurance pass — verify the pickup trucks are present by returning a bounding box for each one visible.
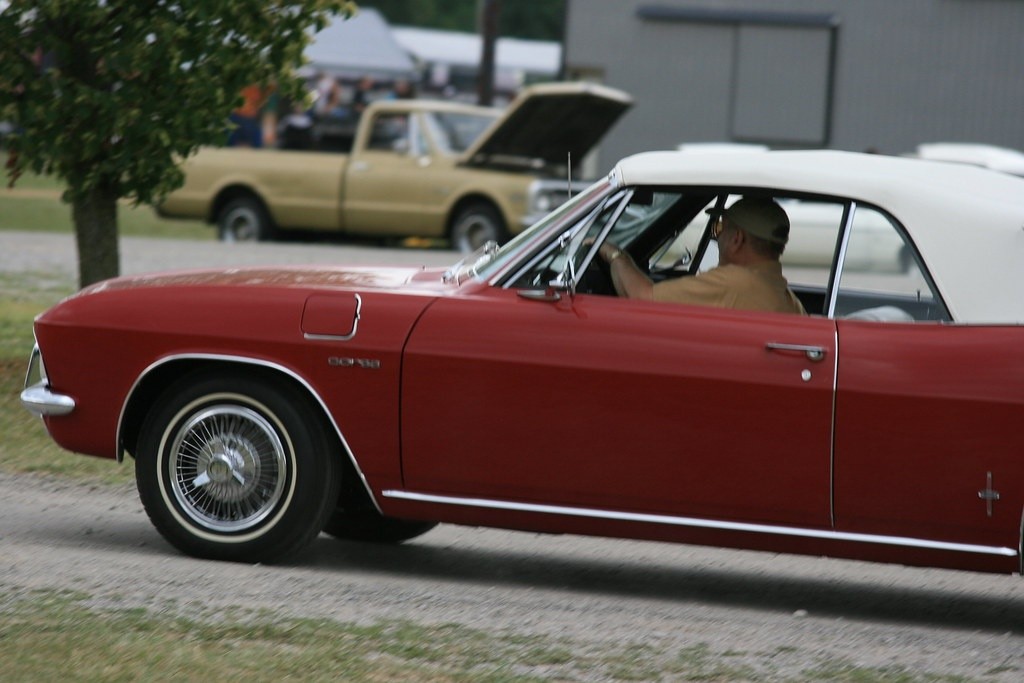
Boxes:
[145,80,637,255]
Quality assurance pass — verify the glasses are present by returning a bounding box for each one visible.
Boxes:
[710,222,737,242]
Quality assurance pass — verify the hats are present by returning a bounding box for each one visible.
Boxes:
[705,196,790,244]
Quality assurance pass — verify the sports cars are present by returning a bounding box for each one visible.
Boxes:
[17,148,1023,563]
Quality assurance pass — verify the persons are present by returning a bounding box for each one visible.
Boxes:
[582,195,807,315]
[221,73,419,148]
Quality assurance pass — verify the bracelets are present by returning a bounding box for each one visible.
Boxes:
[607,248,624,264]
